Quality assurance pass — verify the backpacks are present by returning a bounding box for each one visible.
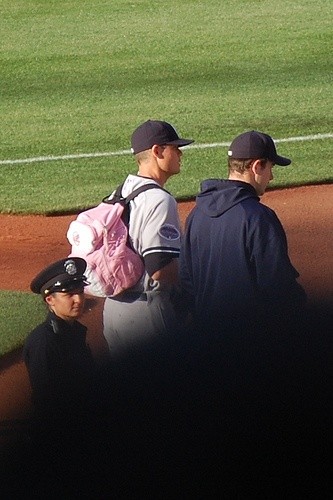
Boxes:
[65,182,173,297]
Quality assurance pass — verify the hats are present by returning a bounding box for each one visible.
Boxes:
[130,119,194,155]
[227,130,292,167]
[30,257,91,299]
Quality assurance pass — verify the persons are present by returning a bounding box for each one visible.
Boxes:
[102,120,195,362]
[177,130,306,332]
[23,258,91,418]
